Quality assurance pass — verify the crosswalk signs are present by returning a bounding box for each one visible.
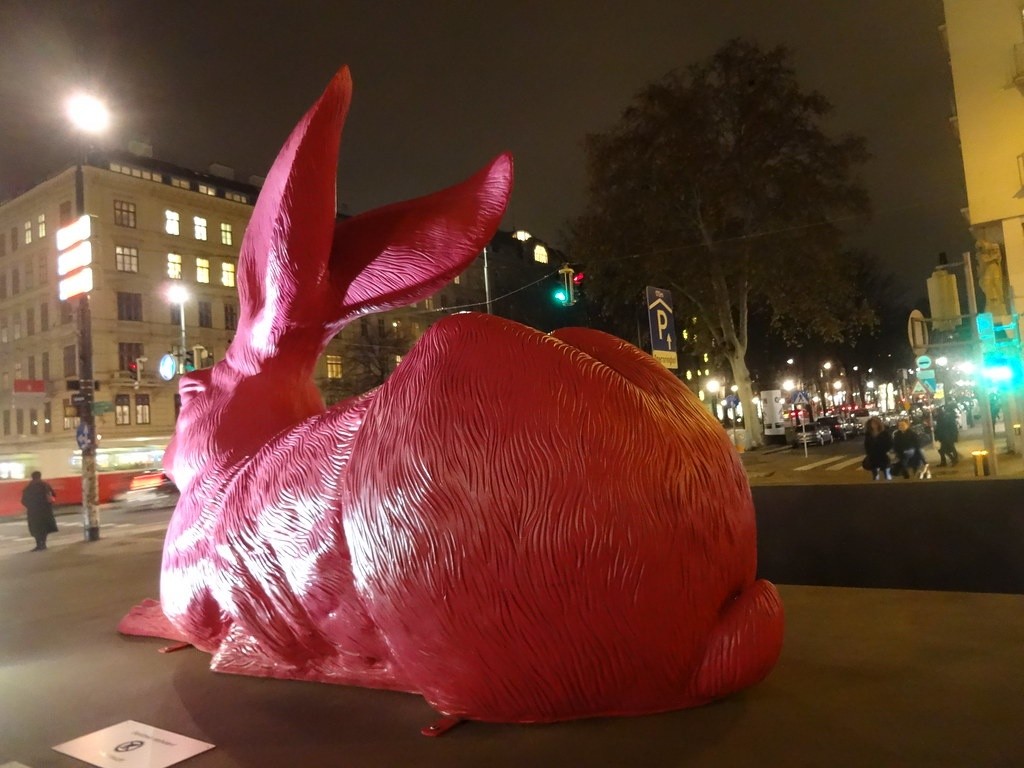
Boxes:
[793,390,809,405]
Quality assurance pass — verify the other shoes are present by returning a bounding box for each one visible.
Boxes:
[33,545,46,552]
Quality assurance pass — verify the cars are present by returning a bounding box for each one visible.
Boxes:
[793,400,932,447]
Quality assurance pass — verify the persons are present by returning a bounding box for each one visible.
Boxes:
[862,416,928,481]
[22,471,60,552]
[933,411,962,468]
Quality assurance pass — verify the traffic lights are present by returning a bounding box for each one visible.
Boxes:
[554,266,584,305]
[128,360,137,380]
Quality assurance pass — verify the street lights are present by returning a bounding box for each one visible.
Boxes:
[69,89,110,541]
[172,286,187,374]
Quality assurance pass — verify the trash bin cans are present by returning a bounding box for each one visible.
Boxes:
[971,451,989,477]
